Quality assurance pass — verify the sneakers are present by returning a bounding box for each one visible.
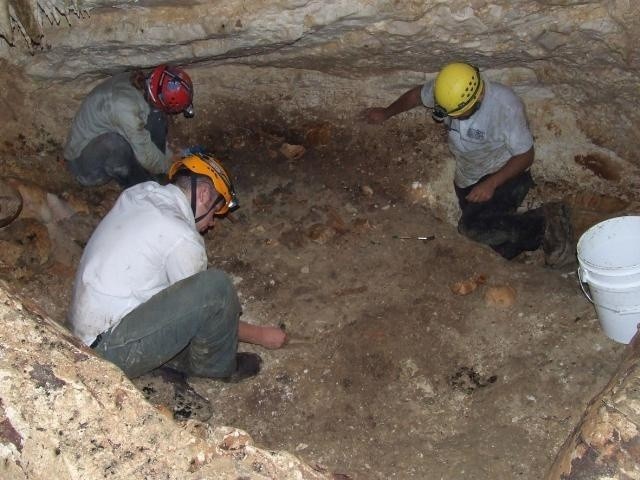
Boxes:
[164,381,215,423]
[209,350,263,385]
[535,200,575,270]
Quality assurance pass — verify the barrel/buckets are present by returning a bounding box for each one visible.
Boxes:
[576,216,640,344]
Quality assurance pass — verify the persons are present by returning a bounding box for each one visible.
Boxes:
[357,62,556,261]
[71,152,291,384]
[61,64,195,214]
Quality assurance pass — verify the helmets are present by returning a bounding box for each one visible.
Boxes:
[432,61,484,123]
[147,64,195,119]
[167,150,238,216]
